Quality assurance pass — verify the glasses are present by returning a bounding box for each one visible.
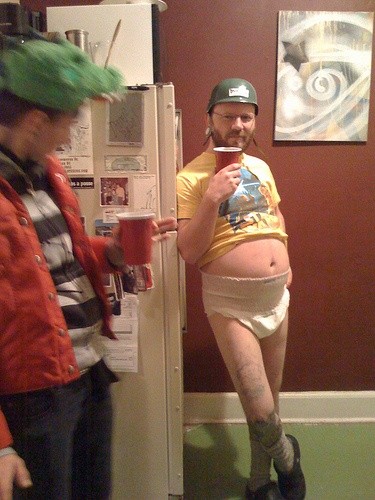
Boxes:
[210,111,256,123]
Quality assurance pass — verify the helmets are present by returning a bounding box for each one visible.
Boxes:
[206,79,258,116]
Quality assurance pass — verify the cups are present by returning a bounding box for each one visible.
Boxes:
[116,211,156,265]
[64,29,89,55]
[213,146,243,173]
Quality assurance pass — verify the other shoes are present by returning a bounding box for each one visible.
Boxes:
[273,435,306,500]
[246,479,279,500]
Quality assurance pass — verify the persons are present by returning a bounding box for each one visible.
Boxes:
[175,79,306,500]
[0,30,179,500]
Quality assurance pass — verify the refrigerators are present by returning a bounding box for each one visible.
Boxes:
[49,81,184,500]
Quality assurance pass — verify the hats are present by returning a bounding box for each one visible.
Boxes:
[2,39,119,111]
